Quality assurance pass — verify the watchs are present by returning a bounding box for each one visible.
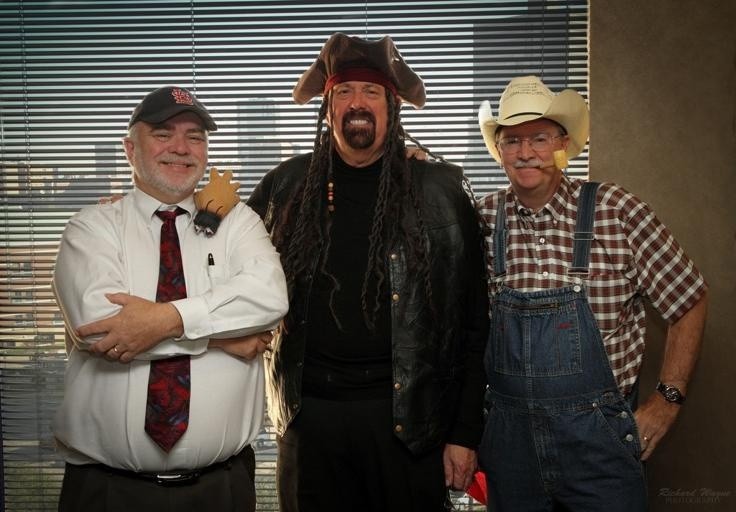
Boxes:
[656,382,686,406]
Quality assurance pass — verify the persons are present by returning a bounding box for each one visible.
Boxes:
[52,87,292,512]
[101,32,488,512]
[406,74,709,512]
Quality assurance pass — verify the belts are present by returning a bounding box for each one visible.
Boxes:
[91,444,251,487]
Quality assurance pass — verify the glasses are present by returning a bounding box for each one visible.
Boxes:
[494,134,562,155]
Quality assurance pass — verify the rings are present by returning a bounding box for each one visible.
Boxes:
[112,346,123,355]
[643,436,651,443]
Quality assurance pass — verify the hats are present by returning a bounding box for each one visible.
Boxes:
[292,32,426,110]
[126,86,217,131]
[477,75,590,161]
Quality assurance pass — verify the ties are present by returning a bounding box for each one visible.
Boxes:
[144,204,191,456]
